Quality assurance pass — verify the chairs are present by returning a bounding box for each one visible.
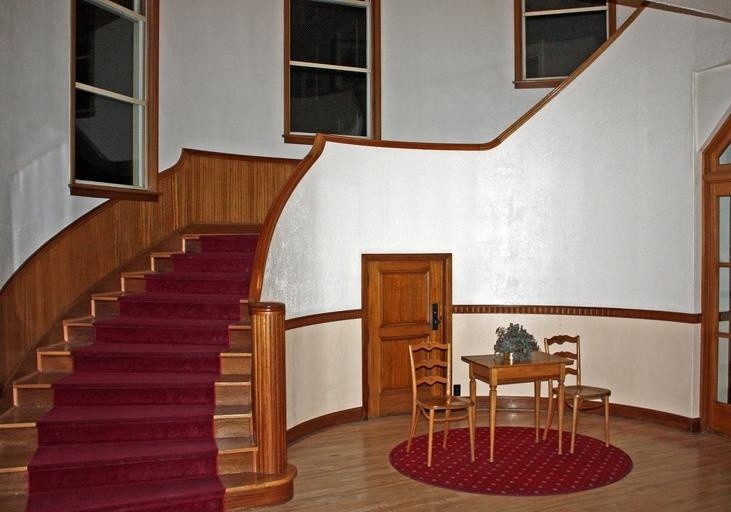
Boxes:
[544,336,612,454]
[406,342,475,468]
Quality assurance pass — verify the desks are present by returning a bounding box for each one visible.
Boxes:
[461,353,572,461]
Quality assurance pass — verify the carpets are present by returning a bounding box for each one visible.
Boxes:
[389,427,632,496]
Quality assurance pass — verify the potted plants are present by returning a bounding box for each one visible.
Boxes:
[493,323,539,360]
